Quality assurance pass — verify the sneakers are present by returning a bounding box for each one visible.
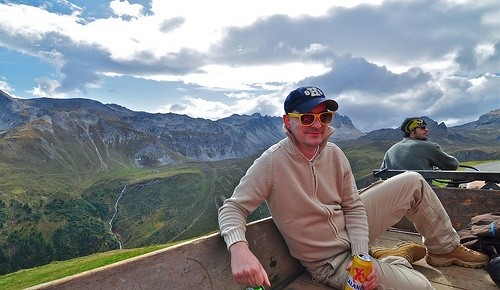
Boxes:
[426,243,489,267]
[368,240,426,264]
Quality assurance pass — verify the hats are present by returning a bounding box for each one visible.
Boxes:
[401,116,427,135]
[284,86,339,115]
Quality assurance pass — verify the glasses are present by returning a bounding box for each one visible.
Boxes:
[288,112,335,126]
[417,126,426,130]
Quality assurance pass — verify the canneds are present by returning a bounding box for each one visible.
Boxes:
[345,252,372,290]
[243,285,266,290]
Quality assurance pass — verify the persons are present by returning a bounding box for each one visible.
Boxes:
[381,117,460,184]
[218,87,491,290]
